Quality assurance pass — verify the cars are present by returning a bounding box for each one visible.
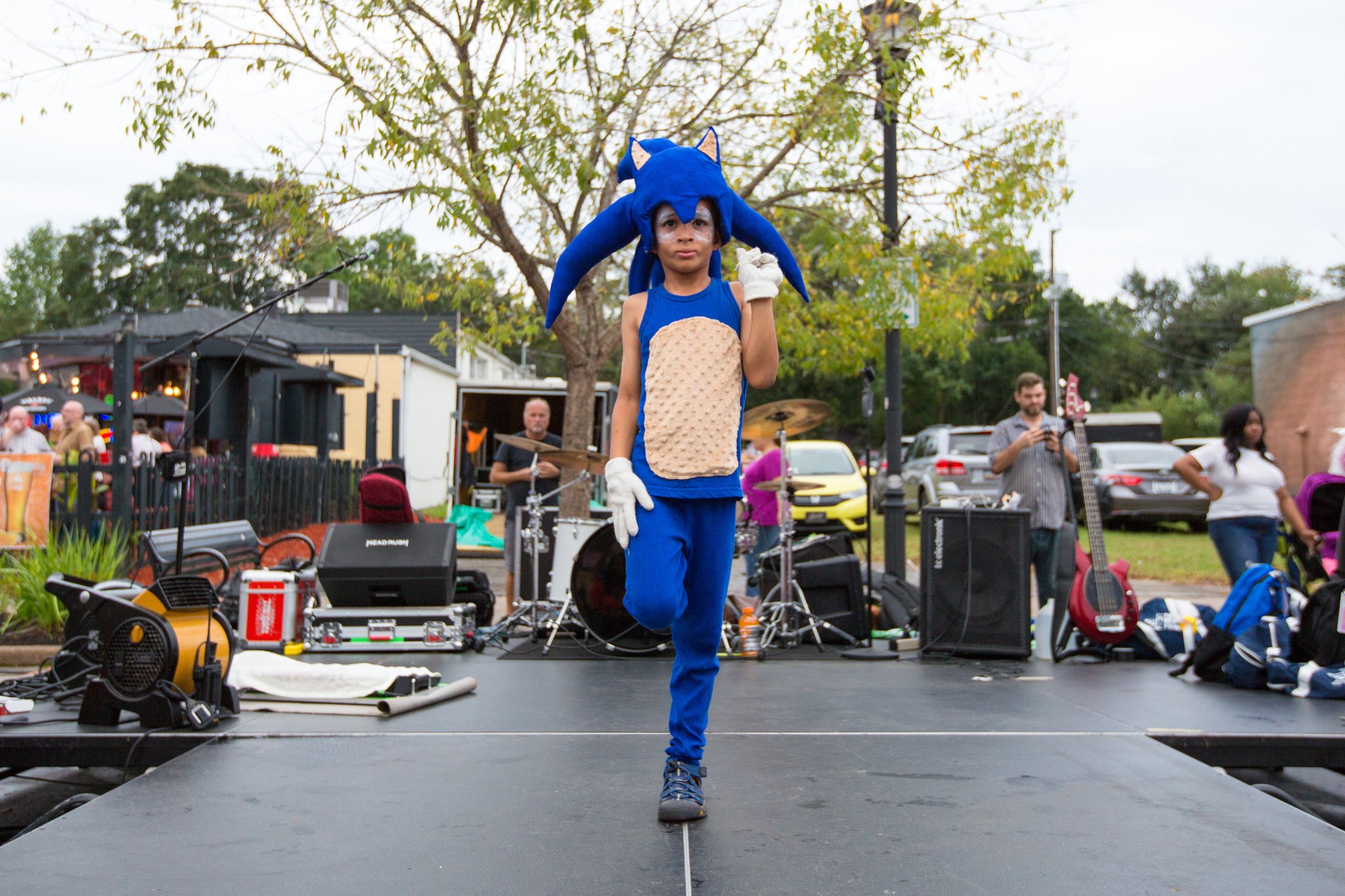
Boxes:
[1070,440,1211,533]
[857,434,917,509]
[785,439,878,541]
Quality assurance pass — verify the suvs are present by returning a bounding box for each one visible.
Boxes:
[903,423,1004,516]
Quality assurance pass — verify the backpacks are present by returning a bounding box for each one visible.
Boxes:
[1130,597,1219,660]
[1170,564,1291,689]
[1297,573,1344,665]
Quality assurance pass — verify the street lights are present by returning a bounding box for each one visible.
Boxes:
[860,0,921,628]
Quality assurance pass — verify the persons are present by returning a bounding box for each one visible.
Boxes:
[545,126,811,822]
[987,371,1081,611]
[488,397,564,615]
[740,435,790,599]
[1173,403,1318,591]
[0,398,206,536]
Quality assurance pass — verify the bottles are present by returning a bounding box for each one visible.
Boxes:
[1036,598,1077,660]
[738,607,761,657]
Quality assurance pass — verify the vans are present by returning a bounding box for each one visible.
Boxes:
[1081,410,1166,443]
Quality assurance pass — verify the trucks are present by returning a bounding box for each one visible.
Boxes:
[398,345,620,560]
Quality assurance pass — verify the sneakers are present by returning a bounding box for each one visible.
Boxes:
[659,757,706,818]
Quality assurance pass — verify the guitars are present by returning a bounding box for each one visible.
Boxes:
[1058,371,1142,646]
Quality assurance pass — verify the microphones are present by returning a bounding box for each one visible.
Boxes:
[360,240,380,261]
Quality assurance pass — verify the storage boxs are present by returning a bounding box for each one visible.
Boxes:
[303,603,476,652]
[237,567,317,650]
[472,489,501,513]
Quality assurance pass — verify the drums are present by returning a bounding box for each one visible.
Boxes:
[548,518,605,604]
[735,490,749,523]
[734,520,761,554]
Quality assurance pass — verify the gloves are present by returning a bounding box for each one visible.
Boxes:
[604,457,654,548]
[735,247,783,303]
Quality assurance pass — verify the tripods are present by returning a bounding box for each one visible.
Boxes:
[755,413,859,652]
[476,449,586,655]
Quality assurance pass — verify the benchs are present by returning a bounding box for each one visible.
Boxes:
[138,520,316,630]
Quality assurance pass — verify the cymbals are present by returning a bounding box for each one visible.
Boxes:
[570,517,670,653]
[537,449,610,475]
[753,476,826,491]
[740,398,831,441]
[493,433,560,453]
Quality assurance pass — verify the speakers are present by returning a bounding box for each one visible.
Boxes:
[316,523,459,607]
[920,502,1030,657]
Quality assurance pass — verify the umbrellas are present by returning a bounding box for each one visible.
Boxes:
[70,393,114,414]
[133,390,186,429]
[1,385,69,415]
